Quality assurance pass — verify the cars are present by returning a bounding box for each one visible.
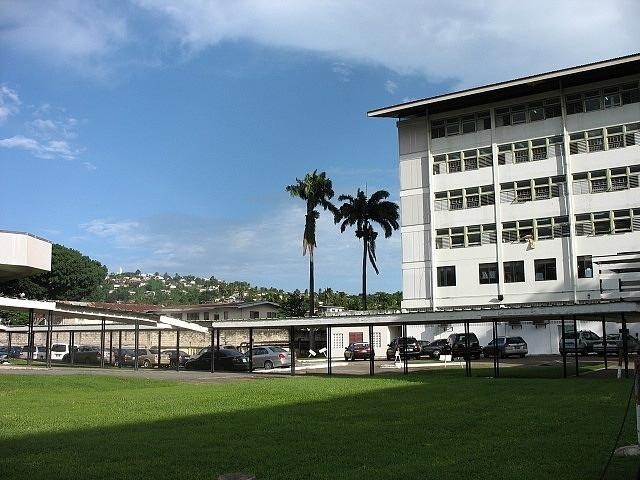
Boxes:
[483,336,528,359]
[422,338,483,359]
[184,349,249,372]
[344,342,374,361]
[418,341,430,347]
[593,332,640,356]
[0,344,194,368]
[243,346,297,370]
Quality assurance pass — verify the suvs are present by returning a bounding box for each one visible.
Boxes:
[386,336,421,360]
[446,332,481,360]
[559,330,600,357]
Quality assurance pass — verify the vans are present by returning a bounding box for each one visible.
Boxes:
[189,345,237,359]
